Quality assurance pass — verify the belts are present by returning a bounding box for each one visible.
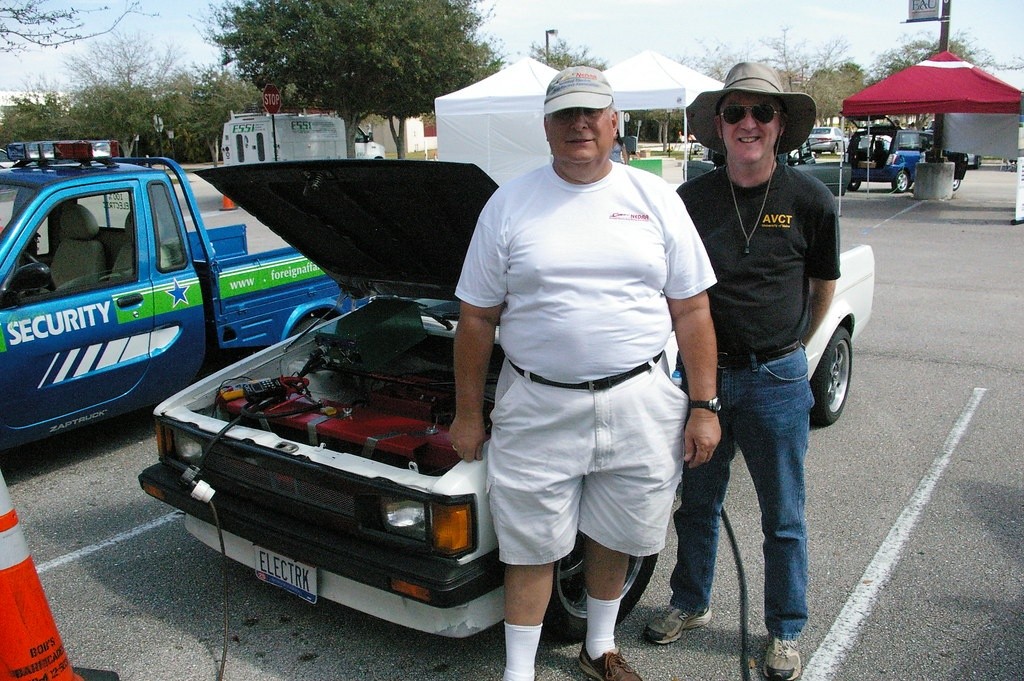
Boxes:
[509,349,665,391]
[717,340,804,369]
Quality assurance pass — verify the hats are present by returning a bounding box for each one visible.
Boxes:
[544,65,614,115]
[686,62,818,155]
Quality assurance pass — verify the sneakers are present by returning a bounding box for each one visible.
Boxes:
[643,604,712,644]
[578,640,643,681]
[762,631,801,681]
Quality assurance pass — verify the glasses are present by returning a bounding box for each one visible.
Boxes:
[720,103,779,124]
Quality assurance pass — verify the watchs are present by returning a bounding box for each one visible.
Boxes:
[689,396,721,411]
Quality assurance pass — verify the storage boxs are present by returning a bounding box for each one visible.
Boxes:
[858,161,875,168]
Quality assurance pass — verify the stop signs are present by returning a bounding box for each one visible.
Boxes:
[264,83,281,114]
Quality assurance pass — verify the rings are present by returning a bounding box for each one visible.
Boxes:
[452,445,457,450]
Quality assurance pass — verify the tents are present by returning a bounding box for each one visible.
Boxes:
[839,52,1024,226]
[601,50,726,184]
[434,55,562,189]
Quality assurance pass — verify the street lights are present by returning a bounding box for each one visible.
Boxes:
[546,29,559,64]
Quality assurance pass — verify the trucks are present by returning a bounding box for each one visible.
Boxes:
[220,109,386,166]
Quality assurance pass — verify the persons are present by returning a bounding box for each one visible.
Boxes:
[609,129,628,165]
[449,66,721,681]
[643,62,841,681]
[894,119,917,130]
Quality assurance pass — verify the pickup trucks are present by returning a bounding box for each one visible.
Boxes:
[0,137,371,456]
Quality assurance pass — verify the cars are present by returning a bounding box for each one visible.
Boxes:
[841,124,969,193]
[808,126,850,153]
[786,139,852,197]
[692,142,718,162]
[966,151,983,170]
[922,119,936,134]
[137,159,874,646]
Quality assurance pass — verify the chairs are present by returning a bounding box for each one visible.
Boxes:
[50,203,104,289]
[108,209,169,284]
[874,140,888,159]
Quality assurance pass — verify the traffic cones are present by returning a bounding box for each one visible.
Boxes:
[218,192,238,212]
[0,470,121,681]
